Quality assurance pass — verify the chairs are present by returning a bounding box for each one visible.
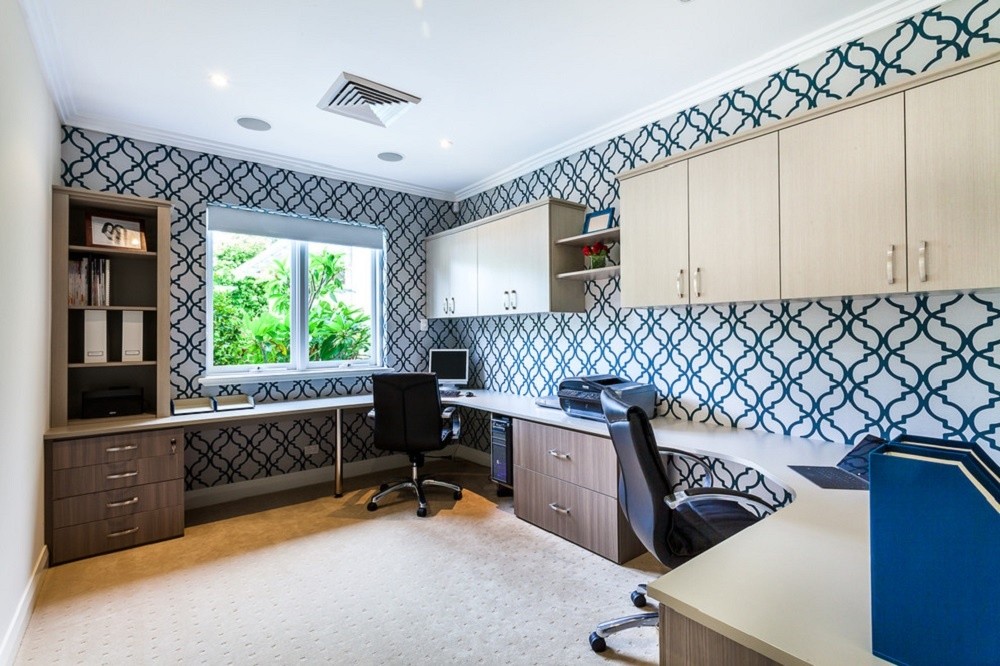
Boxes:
[367,372,461,517]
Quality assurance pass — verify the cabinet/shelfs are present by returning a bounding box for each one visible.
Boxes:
[425,197,586,319]
[49,426,184,565]
[512,418,669,564]
[555,226,621,281]
[50,185,173,428]
[613,43,1000,308]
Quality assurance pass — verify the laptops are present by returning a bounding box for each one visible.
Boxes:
[788,434,890,490]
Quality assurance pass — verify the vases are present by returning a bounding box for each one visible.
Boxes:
[585,256,606,269]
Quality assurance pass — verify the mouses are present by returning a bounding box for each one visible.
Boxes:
[465,392,473,397]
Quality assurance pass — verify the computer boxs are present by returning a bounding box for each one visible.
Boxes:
[491,416,512,484]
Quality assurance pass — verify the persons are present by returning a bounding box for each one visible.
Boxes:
[102,222,125,240]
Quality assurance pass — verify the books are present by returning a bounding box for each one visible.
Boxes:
[68,258,110,307]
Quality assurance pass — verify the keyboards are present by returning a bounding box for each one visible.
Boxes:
[440,390,460,397]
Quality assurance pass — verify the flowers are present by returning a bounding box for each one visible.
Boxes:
[581,242,608,256]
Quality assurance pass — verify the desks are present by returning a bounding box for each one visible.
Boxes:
[45,386,894,666]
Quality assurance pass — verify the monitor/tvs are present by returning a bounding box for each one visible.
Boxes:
[429,349,468,390]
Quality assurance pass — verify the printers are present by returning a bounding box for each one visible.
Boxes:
[556,374,657,422]
